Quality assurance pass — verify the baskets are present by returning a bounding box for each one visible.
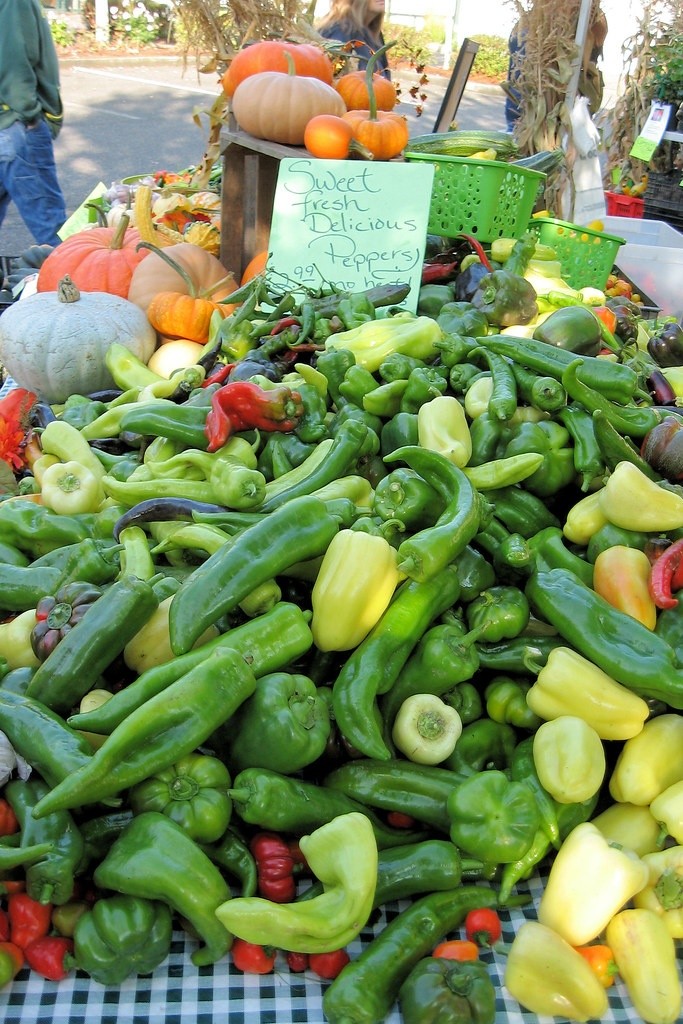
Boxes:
[527,218,626,291]
[605,191,643,218]
[405,152,547,244]
[643,172,683,234]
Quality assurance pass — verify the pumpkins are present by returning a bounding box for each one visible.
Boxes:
[222,31,408,161]
[0,185,272,403]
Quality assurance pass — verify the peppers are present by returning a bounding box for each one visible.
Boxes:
[0,221,683,1024]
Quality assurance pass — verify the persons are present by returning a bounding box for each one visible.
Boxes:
[505,13,529,133]
[317,0,391,80]
[0,0,68,248]
